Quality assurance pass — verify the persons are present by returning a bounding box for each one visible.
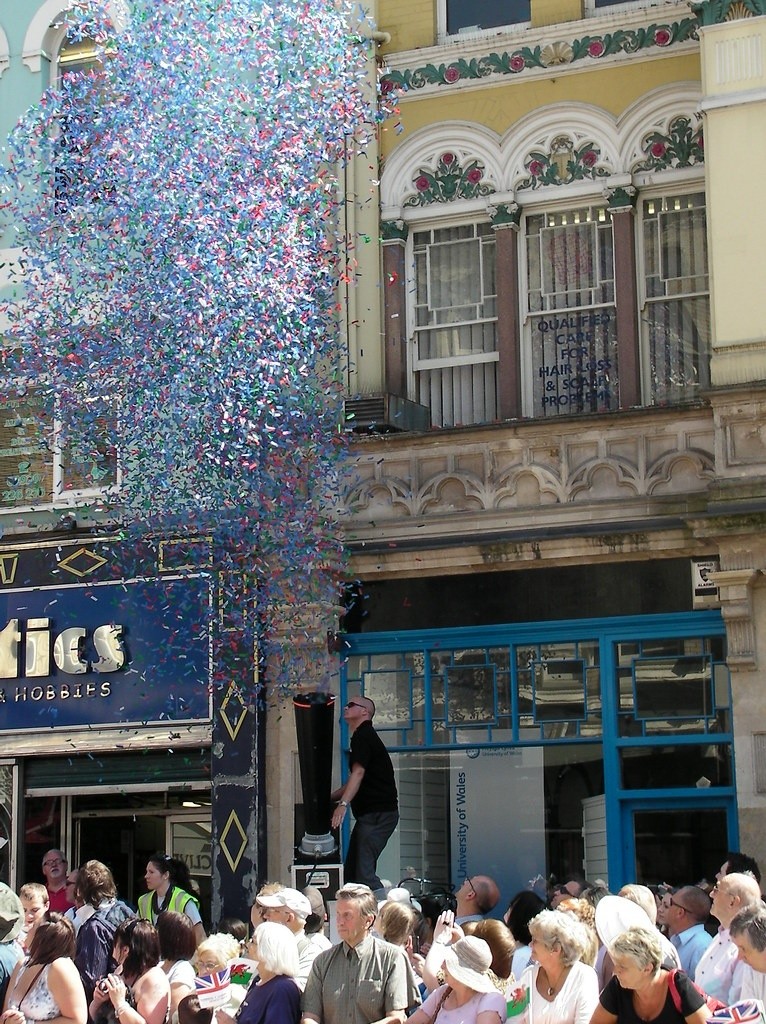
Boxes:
[331,696,400,900]
[0,848,766,1024]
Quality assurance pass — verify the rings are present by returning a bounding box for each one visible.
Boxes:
[444,921,449,925]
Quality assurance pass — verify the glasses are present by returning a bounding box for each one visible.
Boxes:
[560,886,578,898]
[123,918,150,944]
[43,858,67,866]
[248,937,256,945]
[467,876,477,897]
[670,898,692,914]
[345,702,367,710]
[252,897,262,907]
[714,885,734,898]
[65,880,76,887]
[194,960,220,972]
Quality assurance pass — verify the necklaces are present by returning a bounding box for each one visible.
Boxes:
[544,965,566,995]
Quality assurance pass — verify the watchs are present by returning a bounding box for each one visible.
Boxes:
[341,801,347,806]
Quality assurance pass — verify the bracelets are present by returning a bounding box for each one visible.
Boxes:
[435,940,446,945]
[114,1002,130,1018]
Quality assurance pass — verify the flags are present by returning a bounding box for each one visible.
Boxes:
[506,971,531,1019]
[227,959,260,985]
[706,998,766,1024]
[194,967,231,1009]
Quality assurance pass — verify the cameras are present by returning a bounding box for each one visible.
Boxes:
[98,978,111,993]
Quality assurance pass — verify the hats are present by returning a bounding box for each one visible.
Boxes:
[255,888,312,919]
[0,882,25,943]
[442,934,503,994]
[377,888,422,914]
[595,896,661,965]
[302,886,325,933]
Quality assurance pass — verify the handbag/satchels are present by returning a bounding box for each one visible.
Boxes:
[668,968,728,1016]
